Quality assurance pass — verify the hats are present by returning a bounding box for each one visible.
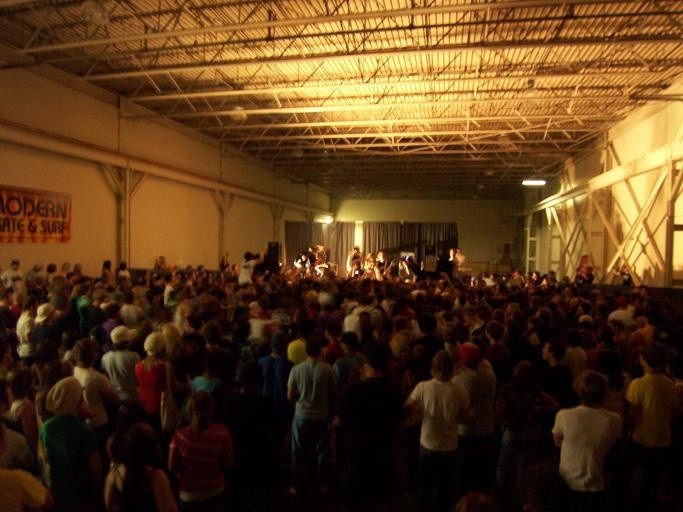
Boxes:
[110,324,138,344]
[33,303,56,323]
[144,331,166,356]
[461,342,481,363]
[45,377,82,411]
[71,340,98,361]
[430,351,451,380]
